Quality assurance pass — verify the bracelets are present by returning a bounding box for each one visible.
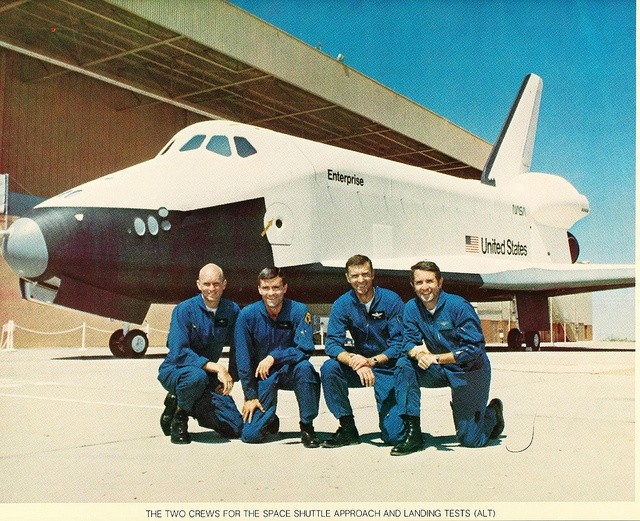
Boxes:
[371,357,379,367]
[433,353,441,365]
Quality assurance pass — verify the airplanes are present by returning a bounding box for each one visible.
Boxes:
[0,73,636,357]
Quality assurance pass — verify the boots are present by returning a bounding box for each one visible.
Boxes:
[487,398,504,437]
[160,392,178,436]
[299,420,319,448]
[320,415,358,447]
[391,416,423,454]
[269,414,279,433]
[171,406,191,443]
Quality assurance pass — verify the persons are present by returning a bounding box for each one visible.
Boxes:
[156,263,244,444]
[390,261,505,457]
[320,254,407,448]
[233,266,322,449]
